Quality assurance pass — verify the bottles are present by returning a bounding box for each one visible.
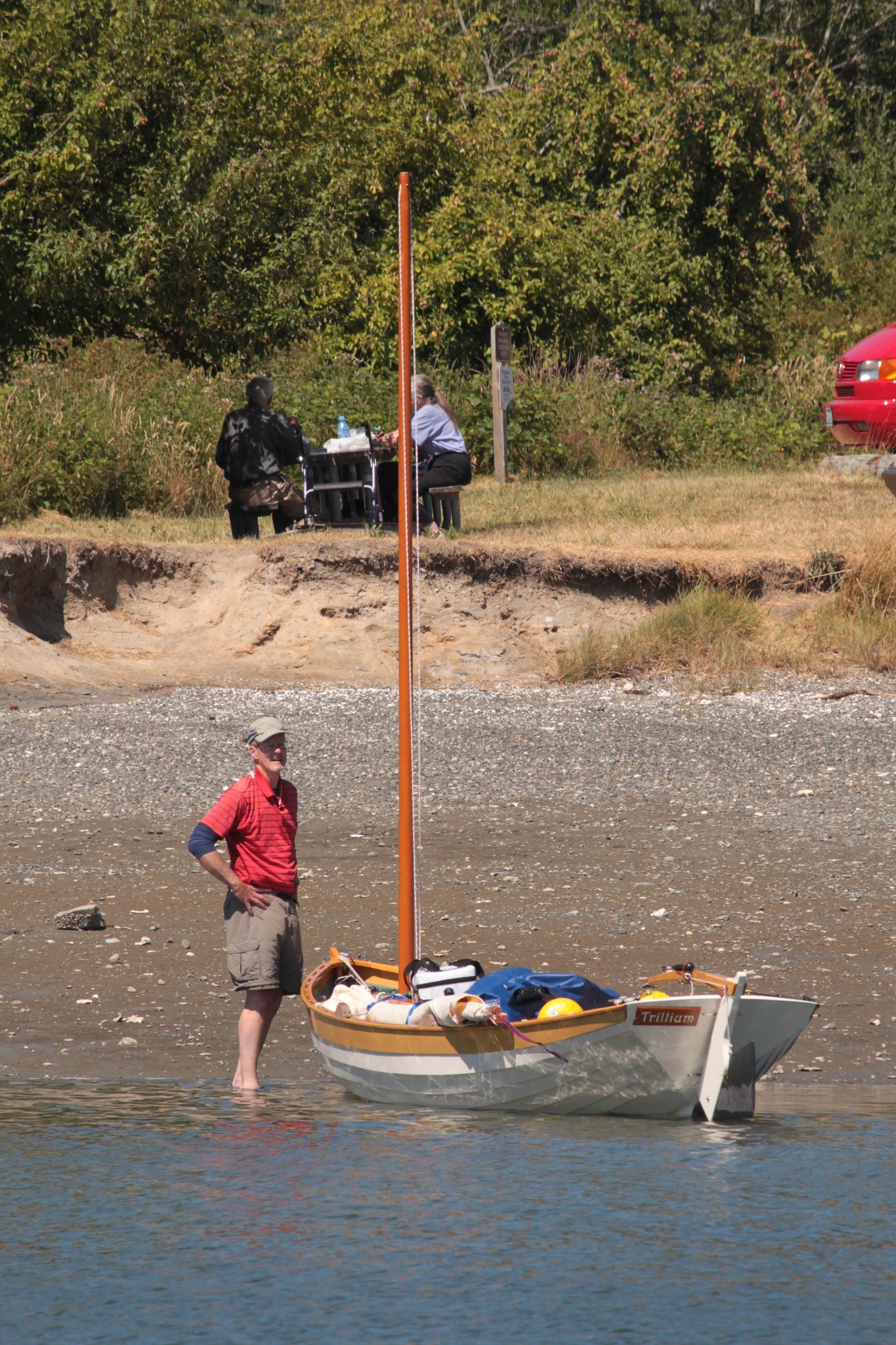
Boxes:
[338,416,349,439]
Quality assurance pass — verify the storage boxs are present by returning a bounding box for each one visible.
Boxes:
[412,964,476,1004]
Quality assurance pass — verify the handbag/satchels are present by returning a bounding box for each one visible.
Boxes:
[404,958,486,1003]
[325,428,377,454]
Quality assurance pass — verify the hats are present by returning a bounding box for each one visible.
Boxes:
[247,717,288,744]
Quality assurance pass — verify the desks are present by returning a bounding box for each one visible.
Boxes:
[302,445,398,531]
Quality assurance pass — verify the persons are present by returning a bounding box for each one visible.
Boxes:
[215,377,306,519]
[187,717,303,1087]
[377,374,470,539]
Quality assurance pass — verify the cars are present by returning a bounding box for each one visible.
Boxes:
[823,320,896,456]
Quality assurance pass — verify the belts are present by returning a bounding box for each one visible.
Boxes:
[231,474,275,488]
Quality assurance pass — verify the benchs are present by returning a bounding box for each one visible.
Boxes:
[423,485,464,533]
[224,502,284,539]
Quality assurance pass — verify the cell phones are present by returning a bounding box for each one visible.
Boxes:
[374,427,385,435]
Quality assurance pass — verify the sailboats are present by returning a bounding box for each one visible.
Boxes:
[297,170,825,1125]
